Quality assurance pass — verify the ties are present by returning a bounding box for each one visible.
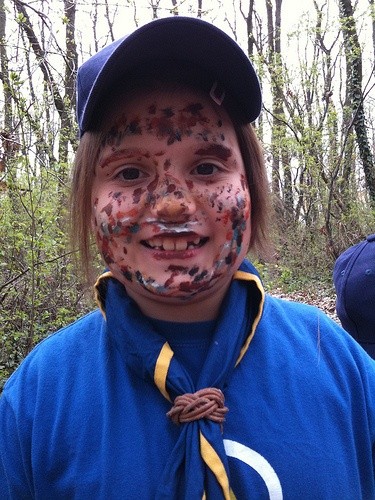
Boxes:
[95,254,266,500]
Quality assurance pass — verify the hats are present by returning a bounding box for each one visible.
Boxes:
[332,234,375,361]
[75,15,261,141]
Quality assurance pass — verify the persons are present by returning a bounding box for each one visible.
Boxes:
[1,16,374,500]
